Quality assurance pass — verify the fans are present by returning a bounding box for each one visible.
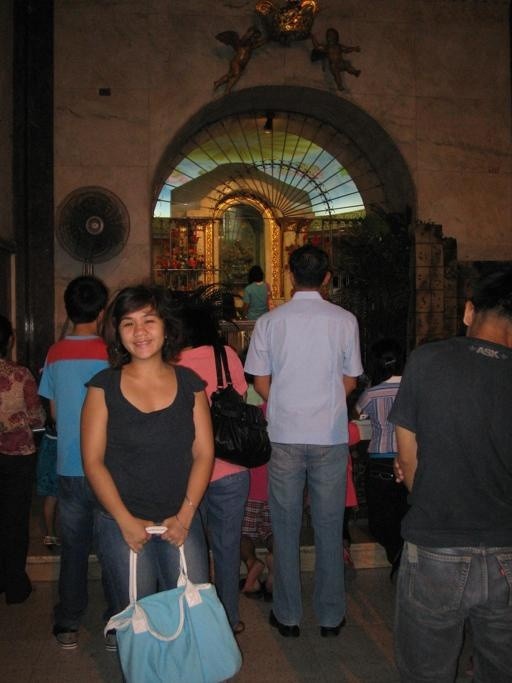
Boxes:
[55,182,131,277]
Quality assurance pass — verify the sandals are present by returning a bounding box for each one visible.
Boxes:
[233,620,245,634]
[344,542,355,580]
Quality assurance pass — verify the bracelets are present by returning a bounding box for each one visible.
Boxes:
[175,513,191,533]
[184,493,198,517]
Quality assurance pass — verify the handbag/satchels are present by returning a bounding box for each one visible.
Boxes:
[104,525,242,683]
[267,283,275,311]
[210,345,270,468]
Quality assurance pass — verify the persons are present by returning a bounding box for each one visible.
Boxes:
[242,265,270,317]
[31,410,64,546]
[352,334,411,582]
[214,19,276,95]
[385,268,511,682]
[309,25,363,91]
[243,244,367,637]
[80,280,216,682]
[172,303,252,634]
[342,419,360,564]
[239,348,275,595]
[35,272,134,652]
[0,316,49,604]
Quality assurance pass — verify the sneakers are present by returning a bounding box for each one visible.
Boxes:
[51,604,79,649]
[106,630,117,652]
[42,534,62,546]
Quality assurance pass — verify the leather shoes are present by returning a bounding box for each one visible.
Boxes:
[319,618,345,637]
[390,548,402,586]
[269,608,299,637]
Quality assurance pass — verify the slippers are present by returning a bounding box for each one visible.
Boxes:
[263,578,273,602]
[240,578,262,598]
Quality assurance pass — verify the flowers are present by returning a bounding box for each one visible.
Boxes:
[156,227,205,288]
